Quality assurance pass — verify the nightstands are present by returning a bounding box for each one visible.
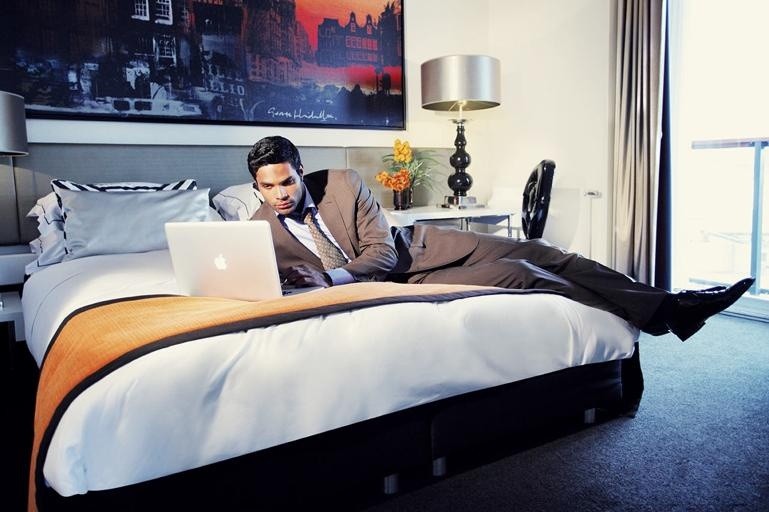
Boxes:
[394,208,513,238]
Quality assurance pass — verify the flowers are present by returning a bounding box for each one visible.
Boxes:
[375,139,442,193]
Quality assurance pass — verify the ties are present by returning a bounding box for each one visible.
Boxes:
[298,208,349,272]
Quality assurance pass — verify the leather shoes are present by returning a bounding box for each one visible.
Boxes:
[671,278,757,343]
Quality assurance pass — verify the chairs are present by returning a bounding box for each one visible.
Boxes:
[521,160,556,241]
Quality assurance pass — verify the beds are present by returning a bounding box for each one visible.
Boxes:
[19,179,643,512]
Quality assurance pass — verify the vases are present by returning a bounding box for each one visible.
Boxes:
[392,191,409,211]
[407,187,415,207]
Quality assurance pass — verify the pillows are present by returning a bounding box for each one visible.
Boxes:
[25,179,263,267]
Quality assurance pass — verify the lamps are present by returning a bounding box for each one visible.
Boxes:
[421,55,503,209]
[1,91,29,300]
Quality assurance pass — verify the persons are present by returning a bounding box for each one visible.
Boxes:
[242,133,757,343]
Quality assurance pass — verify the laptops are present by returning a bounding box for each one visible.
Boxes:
[164,220,326,303]
